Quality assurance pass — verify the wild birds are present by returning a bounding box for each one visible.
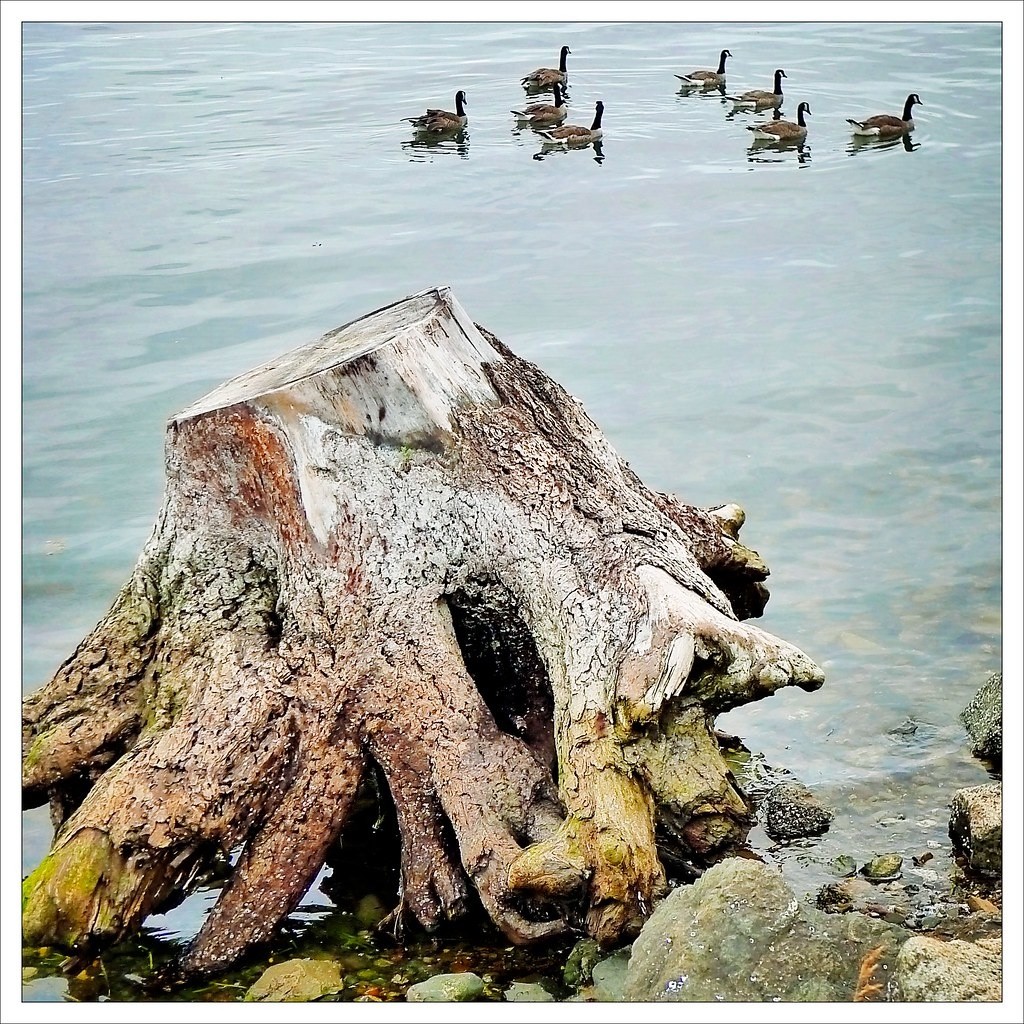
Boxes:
[746,102,812,145]
[400,90,470,135]
[520,45,572,96]
[673,49,733,97]
[725,68,788,114]
[510,80,568,128]
[846,93,923,140]
[531,101,604,148]
